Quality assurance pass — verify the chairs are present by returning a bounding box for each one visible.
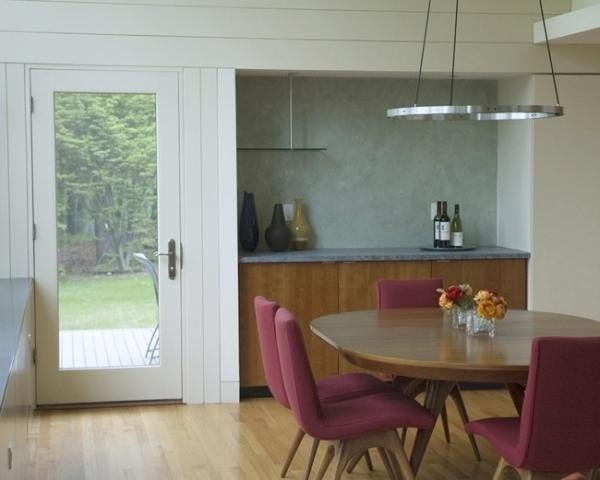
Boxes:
[273,308,435,480]
[254,295,399,480]
[371,277,451,444]
[464,335,599,480]
[132,252,159,365]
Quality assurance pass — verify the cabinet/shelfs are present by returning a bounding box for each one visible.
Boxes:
[339,259,433,391]
[433,258,529,310]
[238,263,339,399]
[0,278,35,480]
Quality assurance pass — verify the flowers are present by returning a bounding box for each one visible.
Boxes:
[435,283,474,311]
[473,289,509,321]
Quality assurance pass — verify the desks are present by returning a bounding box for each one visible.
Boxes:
[309,307,600,479]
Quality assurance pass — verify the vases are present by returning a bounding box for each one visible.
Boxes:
[265,203,291,252]
[465,308,496,338]
[452,306,475,330]
[239,192,259,252]
[288,199,314,249]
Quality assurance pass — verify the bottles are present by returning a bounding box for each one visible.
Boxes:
[434,201,464,248]
